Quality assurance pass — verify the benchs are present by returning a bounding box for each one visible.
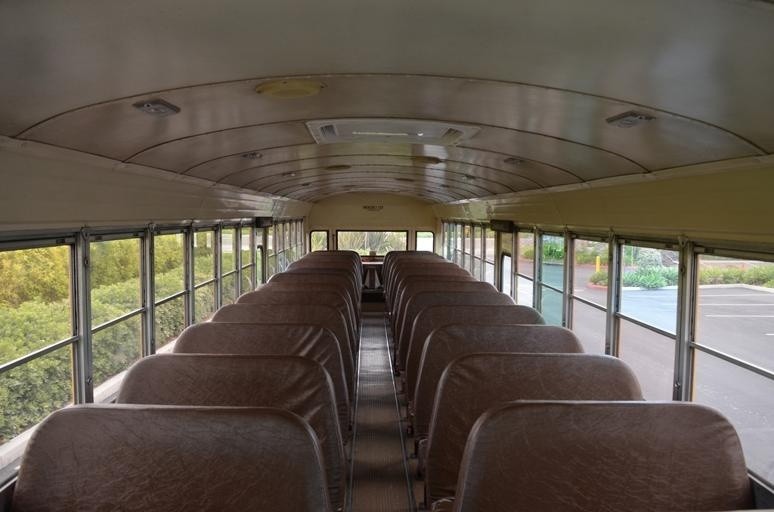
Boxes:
[10,251,358,512]
[384,248,756,511]
